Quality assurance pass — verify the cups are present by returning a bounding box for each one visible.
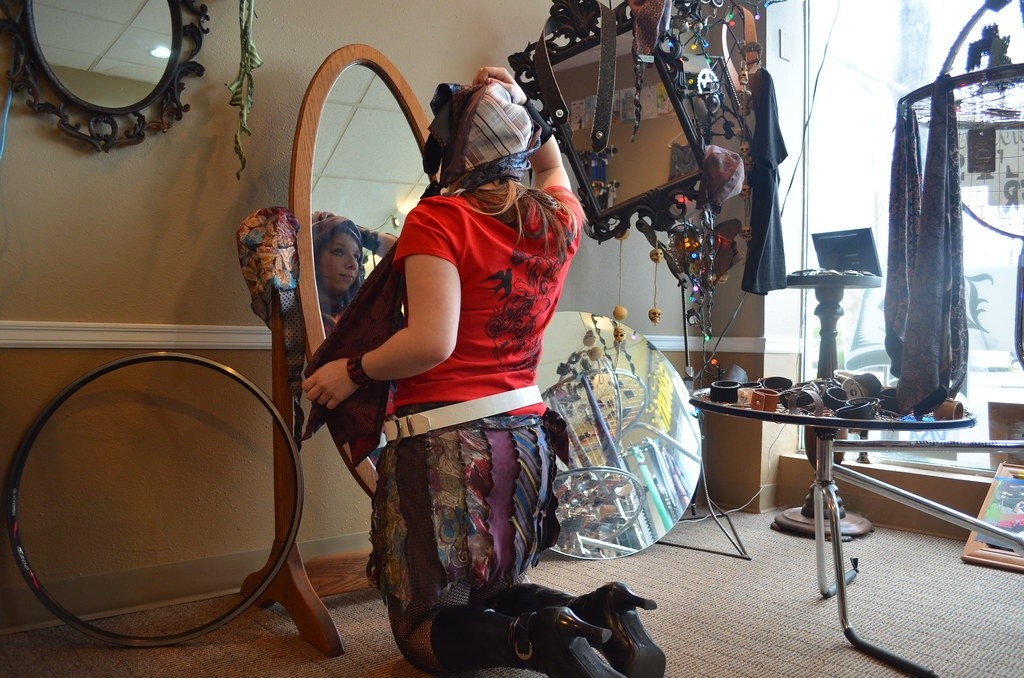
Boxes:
[934,399,963,420]
[552,471,633,551]
[710,370,911,419]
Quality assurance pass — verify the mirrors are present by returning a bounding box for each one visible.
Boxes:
[293,42,447,498]
[507,310,704,562]
[505,0,720,240]
[0,0,212,152]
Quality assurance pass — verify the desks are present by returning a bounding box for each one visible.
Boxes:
[553,465,646,555]
[691,386,1024,677]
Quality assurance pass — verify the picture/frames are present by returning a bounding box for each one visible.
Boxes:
[961,463,1024,574]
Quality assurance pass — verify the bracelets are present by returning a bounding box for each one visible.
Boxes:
[348,353,372,386]
[709,365,965,420]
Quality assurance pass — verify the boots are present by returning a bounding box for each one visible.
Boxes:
[493,582,665,678]
[430,605,629,678]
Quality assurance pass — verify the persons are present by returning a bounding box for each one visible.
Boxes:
[312,210,399,336]
[302,67,667,678]
